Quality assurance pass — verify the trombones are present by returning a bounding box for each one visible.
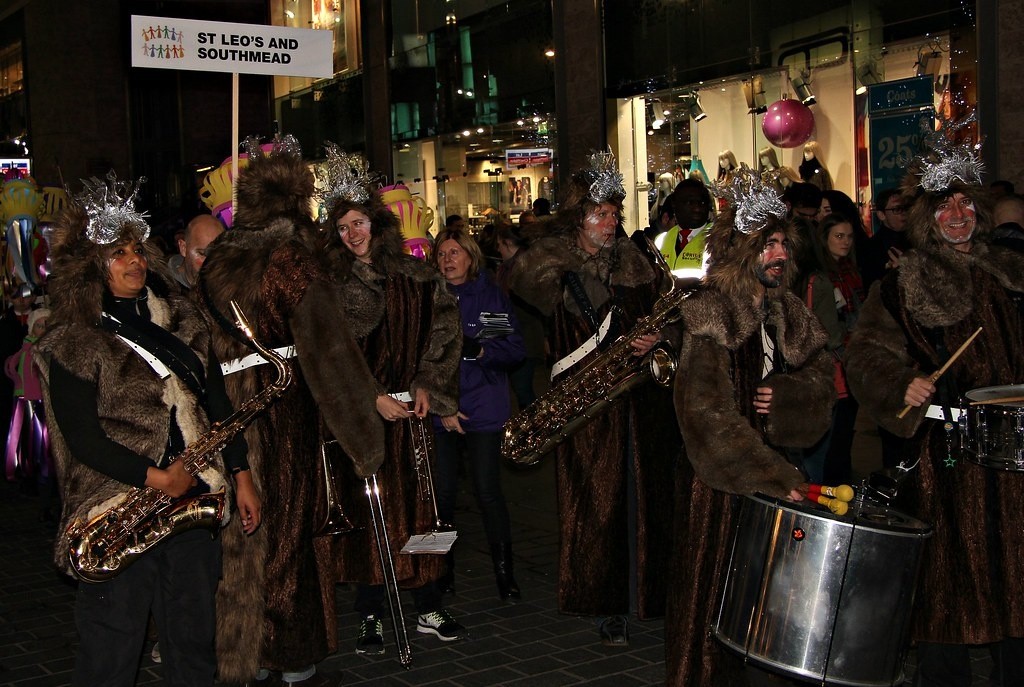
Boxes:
[315,439,415,671]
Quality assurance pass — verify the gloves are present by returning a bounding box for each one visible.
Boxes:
[461,334,481,359]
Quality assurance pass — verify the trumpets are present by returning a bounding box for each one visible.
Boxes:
[405,412,455,532]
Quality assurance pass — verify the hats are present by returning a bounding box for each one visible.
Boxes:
[27,310,51,337]
[703,162,804,292]
[232,132,314,227]
[899,109,1002,256]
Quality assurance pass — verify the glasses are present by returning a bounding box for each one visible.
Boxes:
[33,322,45,330]
[885,206,910,215]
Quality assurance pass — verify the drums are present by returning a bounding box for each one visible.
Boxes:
[708,490,937,687]
[962,383,1024,473]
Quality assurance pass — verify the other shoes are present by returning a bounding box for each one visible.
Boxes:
[152,642,161,663]
[282,672,345,687]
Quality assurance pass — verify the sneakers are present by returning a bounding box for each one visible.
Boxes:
[255,668,270,680]
[417,612,469,642]
[355,615,385,655]
[599,614,630,646]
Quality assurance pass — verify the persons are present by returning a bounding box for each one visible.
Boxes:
[706,150,739,203]
[31,133,1024,687]
[688,169,715,210]
[650,173,675,225]
[513,181,527,207]
[538,175,554,204]
[782,141,833,192]
[755,146,784,187]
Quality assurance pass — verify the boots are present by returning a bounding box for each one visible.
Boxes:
[488,538,522,601]
[437,551,456,593]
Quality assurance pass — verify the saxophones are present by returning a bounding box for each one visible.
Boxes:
[499,229,698,467]
[63,294,296,586]
